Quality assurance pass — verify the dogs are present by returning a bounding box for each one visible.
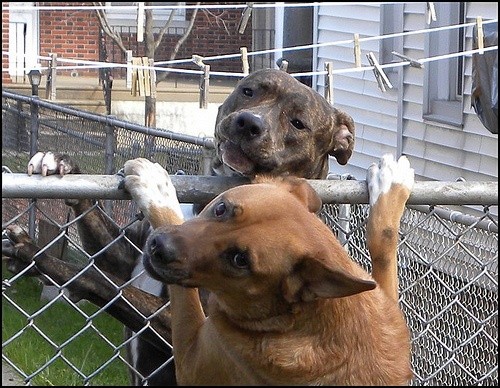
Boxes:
[2,69,356,388]
[117,153,416,388]
